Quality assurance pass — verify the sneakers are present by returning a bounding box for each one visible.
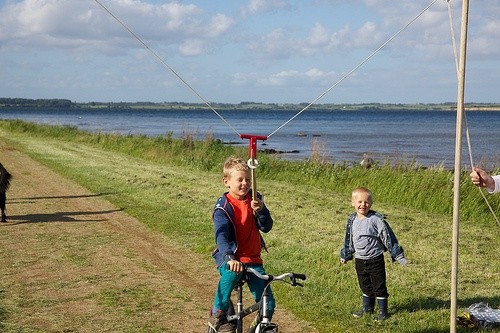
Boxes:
[208,309,234,333]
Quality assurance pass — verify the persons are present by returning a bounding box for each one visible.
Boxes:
[211,154,277,333]
[339,186,408,324]
[469,167,500,194]
[0,163,13,223]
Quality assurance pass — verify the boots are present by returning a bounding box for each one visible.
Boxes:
[352,294,376,317]
[377,298,388,321]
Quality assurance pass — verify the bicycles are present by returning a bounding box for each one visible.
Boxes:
[207,264,306,333]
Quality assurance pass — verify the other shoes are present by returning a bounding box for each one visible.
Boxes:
[0,213,5,222]
